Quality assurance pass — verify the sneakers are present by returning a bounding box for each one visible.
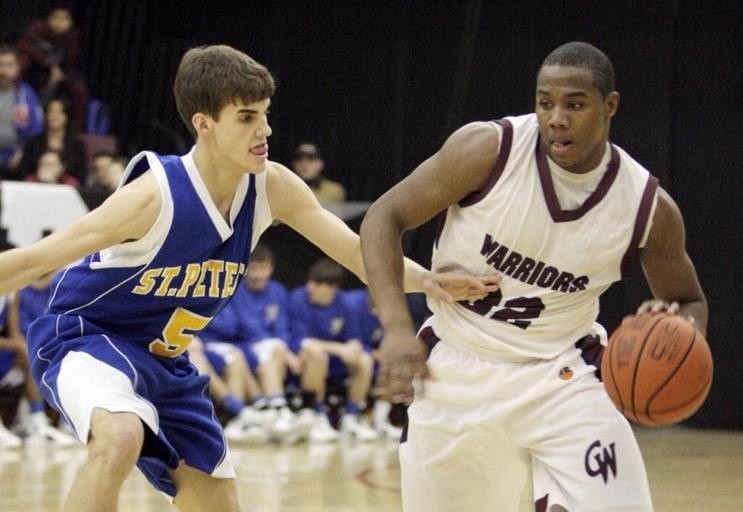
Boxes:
[221,391,406,447]
[0,410,78,450]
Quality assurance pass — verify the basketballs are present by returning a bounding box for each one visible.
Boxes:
[601,314,714,428]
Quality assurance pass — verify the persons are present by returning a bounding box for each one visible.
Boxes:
[1,42,433,447]
[360,39,709,512]
[0,40,501,512]
